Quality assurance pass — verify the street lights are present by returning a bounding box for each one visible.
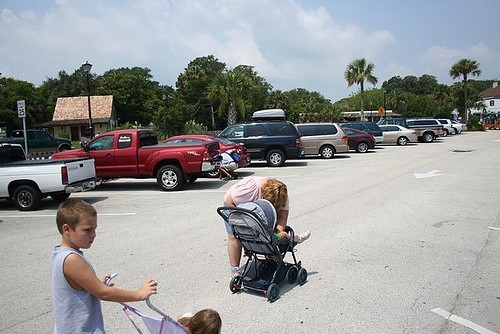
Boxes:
[82,60,93,141]
[383,88,386,117]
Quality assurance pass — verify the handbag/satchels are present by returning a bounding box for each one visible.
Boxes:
[225,151,241,163]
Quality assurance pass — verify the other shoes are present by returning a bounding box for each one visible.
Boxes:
[224,176,232,180]
[230,266,239,278]
[298,230,311,244]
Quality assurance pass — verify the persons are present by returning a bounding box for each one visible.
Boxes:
[273,227,313,245]
[48,197,159,334]
[175,308,222,334]
[221,176,290,278]
[219,152,240,180]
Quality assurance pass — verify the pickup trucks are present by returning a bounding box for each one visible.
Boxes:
[0,143,96,211]
[48,128,223,191]
[0,129,73,154]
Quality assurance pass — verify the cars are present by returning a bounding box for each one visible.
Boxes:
[377,124,418,146]
[160,134,252,178]
[341,127,376,153]
[376,117,468,143]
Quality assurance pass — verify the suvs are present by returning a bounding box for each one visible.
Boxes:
[338,122,384,143]
[294,123,350,160]
[214,109,305,167]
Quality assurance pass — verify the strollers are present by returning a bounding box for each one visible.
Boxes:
[217,198,308,302]
[105,272,193,334]
